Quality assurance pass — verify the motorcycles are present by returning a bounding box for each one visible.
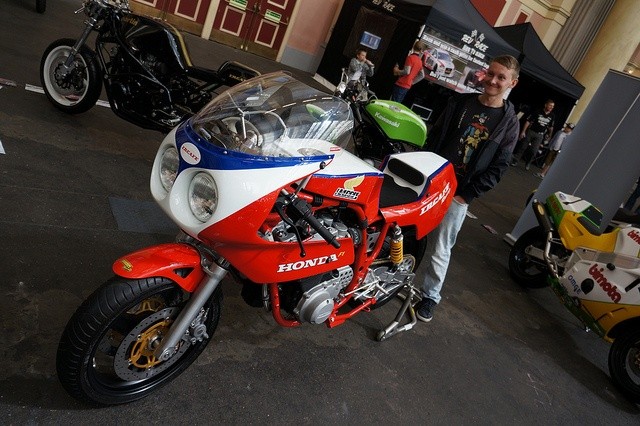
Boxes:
[56,69,459,408]
[508,190,640,403]
[39,0,266,135]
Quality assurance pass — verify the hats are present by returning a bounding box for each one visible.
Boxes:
[564,122,575,130]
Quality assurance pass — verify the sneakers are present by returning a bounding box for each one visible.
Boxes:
[416,298,437,323]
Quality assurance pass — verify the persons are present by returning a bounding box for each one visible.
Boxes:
[390,41,428,103]
[415,53,520,323]
[346,48,374,86]
[534,122,576,179]
[510,98,556,170]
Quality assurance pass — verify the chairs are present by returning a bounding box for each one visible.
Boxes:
[411,102,432,123]
[332,70,369,96]
